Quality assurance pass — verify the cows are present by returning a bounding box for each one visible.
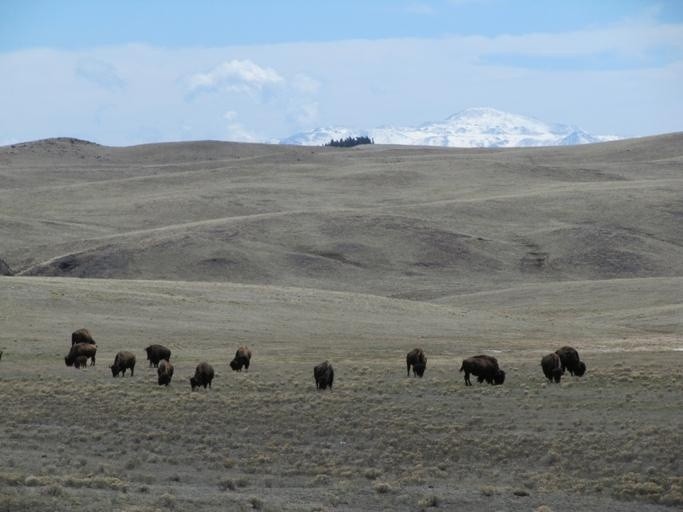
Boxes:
[189,361,215,391]
[64,328,98,369]
[109,351,136,377]
[145,343,175,387]
[540,344,588,382]
[229,346,253,372]
[406,347,428,378]
[456,354,507,386]
[312,359,336,391]
